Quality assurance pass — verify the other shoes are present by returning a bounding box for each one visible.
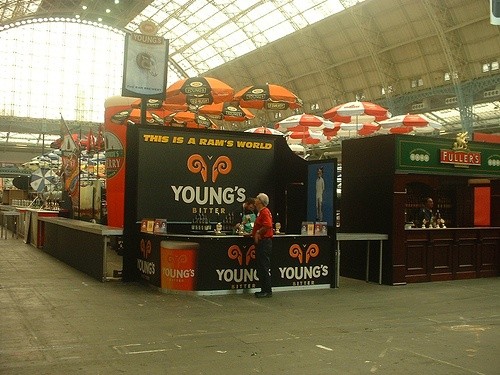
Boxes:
[254,291,273,298]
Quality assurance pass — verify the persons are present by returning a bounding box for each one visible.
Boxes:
[417,197,439,228]
[243,193,272,298]
[315,168,325,221]
[233,197,258,233]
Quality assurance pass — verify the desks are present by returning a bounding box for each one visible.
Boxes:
[0,205,122,283]
[336,232,389,289]
[190,231,234,235]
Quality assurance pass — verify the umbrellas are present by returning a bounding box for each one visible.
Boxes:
[12,75,444,210]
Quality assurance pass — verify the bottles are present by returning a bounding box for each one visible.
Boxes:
[191,211,212,231]
[275,212,281,233]
[422,209,441,226]
[216,212,237,231]
[45,195,60,210]
